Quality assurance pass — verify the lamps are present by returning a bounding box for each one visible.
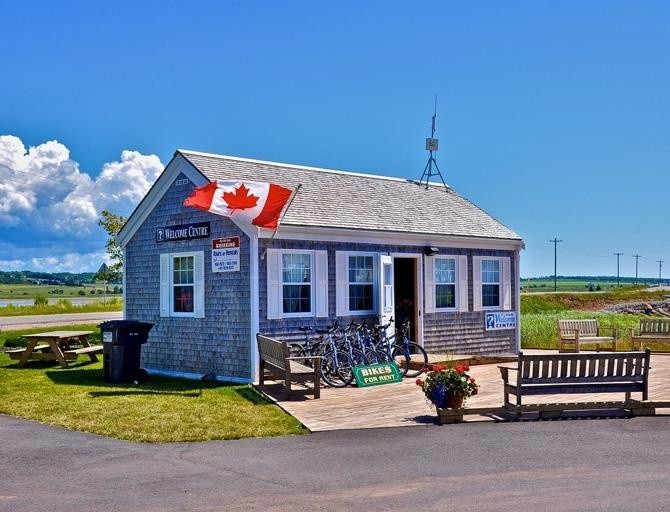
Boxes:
[426,248,440,257]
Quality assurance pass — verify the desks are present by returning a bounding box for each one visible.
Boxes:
[18,330,99,368]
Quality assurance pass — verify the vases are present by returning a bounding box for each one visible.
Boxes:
[437,407,465,424]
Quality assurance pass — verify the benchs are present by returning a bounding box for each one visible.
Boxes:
[628,318,670,349]
[6,343,51,360]
[64,345,104,356]
[557,317,618,354]
[498,347,655,409]
[256,332,325,399]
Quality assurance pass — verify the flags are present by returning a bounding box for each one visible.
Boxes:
[183,179,292,230]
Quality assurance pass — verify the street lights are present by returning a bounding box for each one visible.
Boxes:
[612,252,624,288]
[632,254,642,286]
[656,258,665,283]
[548,238,562,292]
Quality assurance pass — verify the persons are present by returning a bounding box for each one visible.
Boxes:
[394,258,411,356]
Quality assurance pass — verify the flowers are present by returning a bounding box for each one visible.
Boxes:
[416,359,479,409]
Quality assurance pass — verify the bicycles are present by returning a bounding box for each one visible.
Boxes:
[284,316,430,389]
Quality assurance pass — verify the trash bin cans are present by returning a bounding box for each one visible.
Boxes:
[96,320,155,384]
